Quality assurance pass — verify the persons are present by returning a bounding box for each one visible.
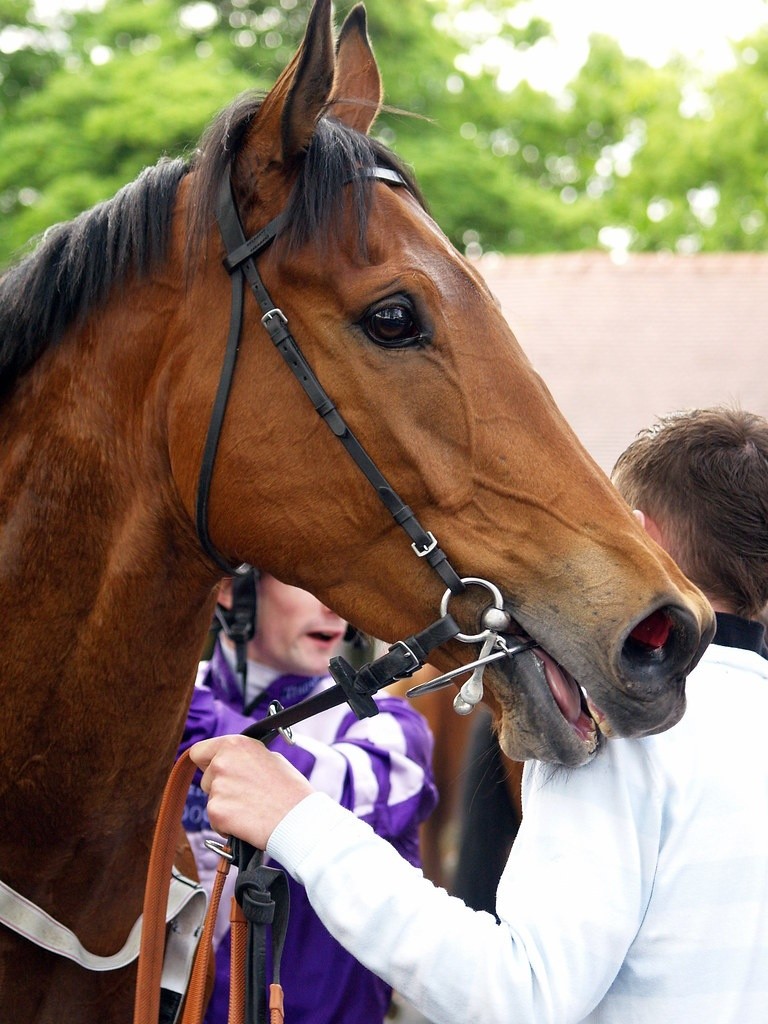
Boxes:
[183,408,768,1024]
[166,564,437,1024]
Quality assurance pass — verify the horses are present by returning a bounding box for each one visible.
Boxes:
[0,0,716,1023]
